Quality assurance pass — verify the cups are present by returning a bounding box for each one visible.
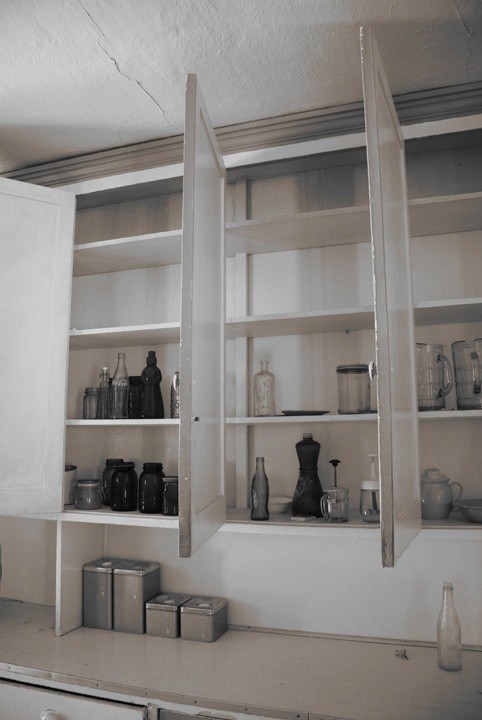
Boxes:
[320,486,350,523]
[64,465,78,504]
[359,479,379,523]
[72,479,103,510]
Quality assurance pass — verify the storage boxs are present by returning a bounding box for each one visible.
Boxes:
[145,593,193,638]
[180,596,227,642]
[114,561,160,634]
[82,556,127,630]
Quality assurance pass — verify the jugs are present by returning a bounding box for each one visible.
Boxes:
[415,342,453,411]
[451,338,482,410]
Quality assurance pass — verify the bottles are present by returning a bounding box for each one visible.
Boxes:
[101,459,123,506]
[110,353,128,419]
[291,432,324,518]
[112,462,138,512]
[171,372,180,419]
[128,376,143,419]
[82,387,98,419]
[163,476,178,516]
[337,365,371,414]
[437,583,462,671]
[250,457,269,520]
[253,360,275,417]
[138,462,165,514]
[98,366,110,419]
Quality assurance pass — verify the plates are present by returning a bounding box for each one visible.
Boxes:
[282,410,329,415]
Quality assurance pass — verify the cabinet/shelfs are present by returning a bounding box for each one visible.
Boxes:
[0,25,482,720]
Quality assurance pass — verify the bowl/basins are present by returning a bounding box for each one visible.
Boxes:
[267,497,293,514]
[453,498,481,523]
